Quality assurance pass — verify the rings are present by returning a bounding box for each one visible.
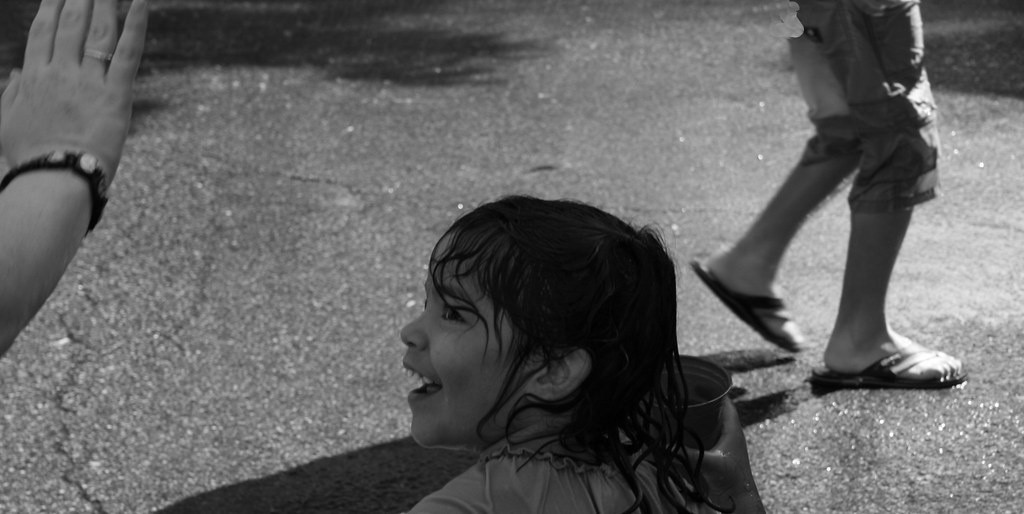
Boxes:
[82,50,112,61]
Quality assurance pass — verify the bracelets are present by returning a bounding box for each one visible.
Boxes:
[0,149,109,235]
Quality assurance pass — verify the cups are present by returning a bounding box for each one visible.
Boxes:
[654,354,733,450]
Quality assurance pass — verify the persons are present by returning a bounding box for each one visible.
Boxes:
[687,0,967,389]
[401,196,729,514]
[0,0,149,356]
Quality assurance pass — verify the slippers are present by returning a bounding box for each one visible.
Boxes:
[692,260,804,352]
[811,344,967,388]
